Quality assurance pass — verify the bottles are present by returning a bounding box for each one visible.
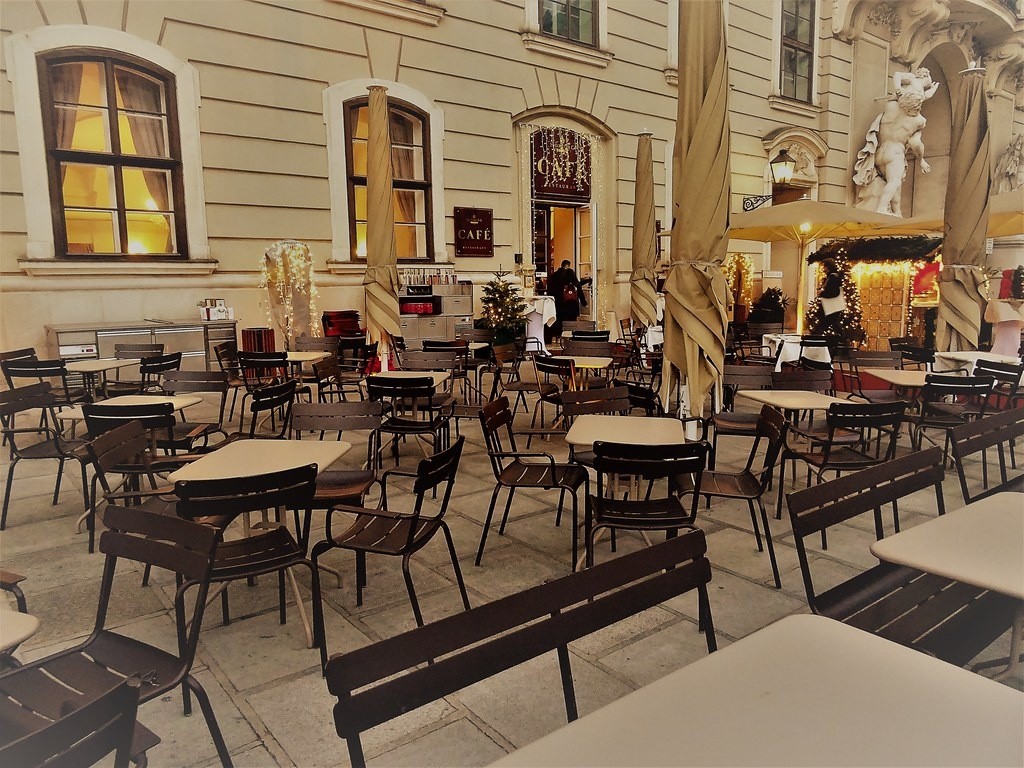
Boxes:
[402,268,457,285]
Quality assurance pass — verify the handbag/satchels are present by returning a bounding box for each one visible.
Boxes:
[563,284,578,301]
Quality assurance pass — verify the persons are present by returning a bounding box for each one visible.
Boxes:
[549,260,588,320]
[875,68,939,217]
[812,262,842,335]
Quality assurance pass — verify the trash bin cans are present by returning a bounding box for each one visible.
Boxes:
[735,303,750,323]
[241,327,281,380]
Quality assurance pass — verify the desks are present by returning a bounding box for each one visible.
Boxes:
[764,330,835,373]
[359,371,450,470]
[560,330,610,340]
[737,385,862,483]
[61,360,141,436]
[870,492,1024,682]
[864,369,971,463]
[935,351,1024,413]
[445,342,489,402]
[564,415,685,572]
[547,356,613,442]
[483,614,1024,768]
[248,351,331,433]
[168,439,352,647]
[56,395,202,534]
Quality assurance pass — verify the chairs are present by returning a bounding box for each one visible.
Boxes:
[0,308,1024,768]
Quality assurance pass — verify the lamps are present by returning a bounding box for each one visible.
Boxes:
[743,149,796,211]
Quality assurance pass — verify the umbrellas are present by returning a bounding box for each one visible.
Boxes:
[658,194,935,334]
[873,189,1024,238]
[937,62,991,403]
[632,128,658,329]
[663,0,730,439]
[363,79,403,372]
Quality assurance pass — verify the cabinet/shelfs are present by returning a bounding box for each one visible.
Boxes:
[398,279,472,347]
[44,315,239,392]
